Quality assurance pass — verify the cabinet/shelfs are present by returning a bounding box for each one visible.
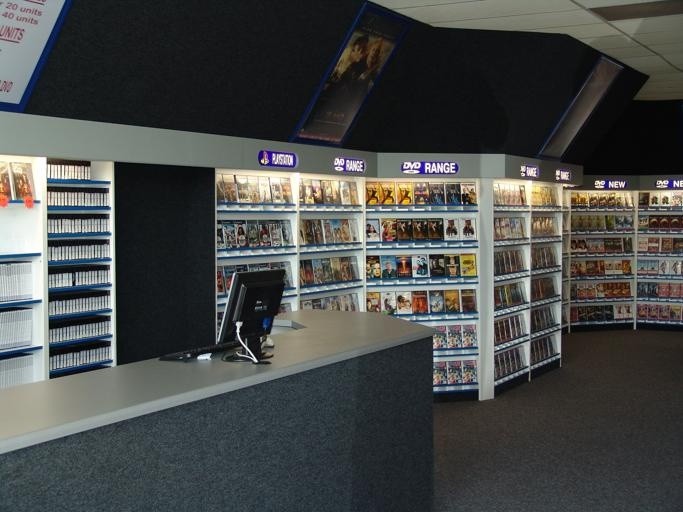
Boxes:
[561,188,683,329]
[480,179,565,399]
[216,169,479,391]
[44,157,117,379]
[0,155,49,389]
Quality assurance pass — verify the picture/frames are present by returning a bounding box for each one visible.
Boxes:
[535,55,624,162]
[287,2,411,148]
[0,0,72,113]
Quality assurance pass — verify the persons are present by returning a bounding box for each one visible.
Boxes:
[339,37,385,94]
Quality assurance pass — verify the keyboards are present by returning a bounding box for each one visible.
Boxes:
[159,338,242,360]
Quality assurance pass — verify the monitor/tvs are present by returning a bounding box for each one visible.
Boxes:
[216,268,285,362]
